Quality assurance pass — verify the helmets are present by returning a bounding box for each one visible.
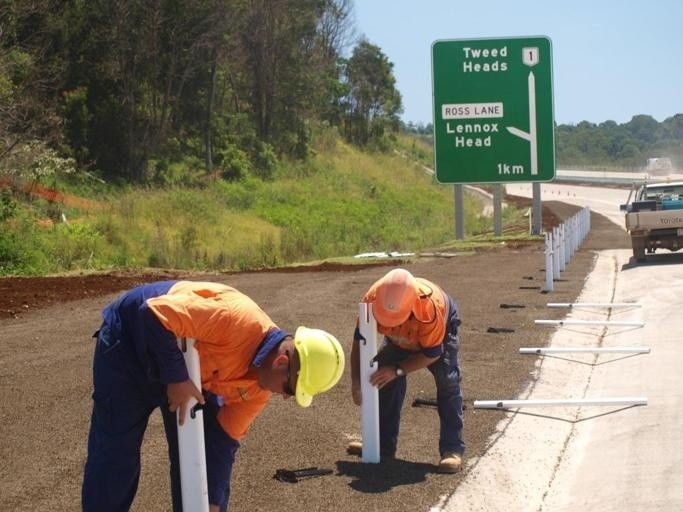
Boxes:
[372,268,417,327]
[294,325,344,409]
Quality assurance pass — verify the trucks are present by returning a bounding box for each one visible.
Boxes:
[646,158,672,175]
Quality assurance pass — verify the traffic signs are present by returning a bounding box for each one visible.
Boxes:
[431,35,556,185]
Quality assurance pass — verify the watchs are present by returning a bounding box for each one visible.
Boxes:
[395,362,404,376]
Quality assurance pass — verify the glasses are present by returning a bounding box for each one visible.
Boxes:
[282,350,294,397]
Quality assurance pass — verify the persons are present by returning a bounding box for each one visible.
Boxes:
[82,280,346,512]
[350,268,466,473]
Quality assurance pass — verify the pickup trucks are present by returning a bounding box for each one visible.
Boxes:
[620,182,683,263]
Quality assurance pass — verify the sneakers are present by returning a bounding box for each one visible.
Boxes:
[439,450,462,473]
[346,442,397,458]
